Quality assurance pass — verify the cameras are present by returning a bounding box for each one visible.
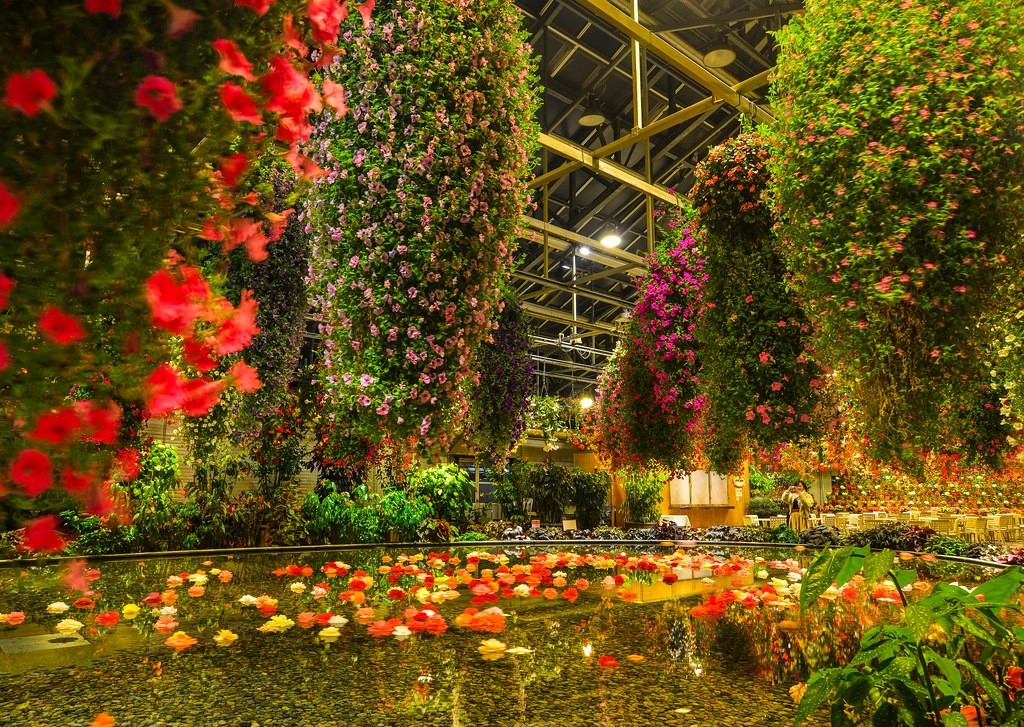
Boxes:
[792,487,797,492]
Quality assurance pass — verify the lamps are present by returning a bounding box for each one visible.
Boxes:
[556,260,573,279]
[577,88,606,127]
[703,30,736,68]
[600,221,621,248]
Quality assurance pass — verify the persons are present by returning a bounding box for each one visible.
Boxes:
[782,480,813,534]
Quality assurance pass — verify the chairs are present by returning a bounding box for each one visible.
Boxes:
[741,509,1024,545]
